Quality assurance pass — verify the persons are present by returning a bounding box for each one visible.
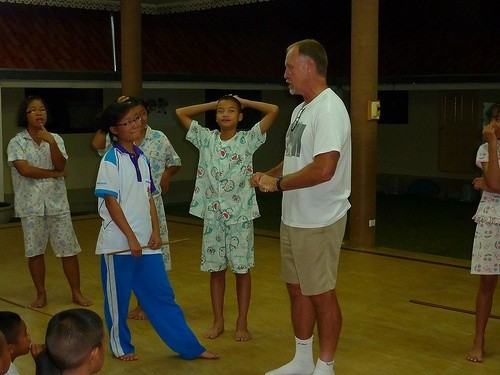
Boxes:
[464,101,500,363]
[36,346,61,375]
[0,311,48,375]
[91,94,182,320]
[94,103,221,361]
[174,93,280,341]
[44,308,106,375]
[0,332,12,375]
[247,38,353,375]
[5,95,94,307]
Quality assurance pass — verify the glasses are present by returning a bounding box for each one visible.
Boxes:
[114,116,140,126]
[25,109,46,115]
[290,105,306,132]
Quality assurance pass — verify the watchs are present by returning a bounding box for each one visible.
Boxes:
[276,176,283,191]
